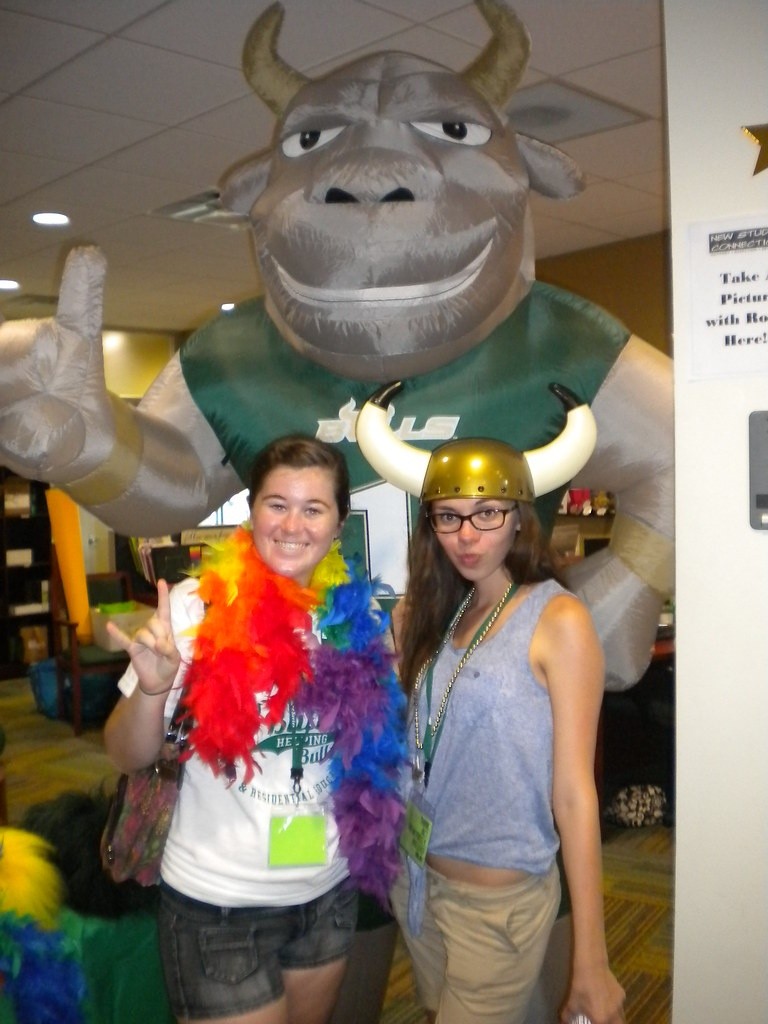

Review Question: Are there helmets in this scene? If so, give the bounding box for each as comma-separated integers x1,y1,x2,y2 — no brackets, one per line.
355,379,597,507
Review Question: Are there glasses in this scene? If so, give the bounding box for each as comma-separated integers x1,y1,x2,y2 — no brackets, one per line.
426,504,519,533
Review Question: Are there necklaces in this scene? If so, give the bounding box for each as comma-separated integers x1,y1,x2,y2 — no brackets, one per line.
413,579,510,750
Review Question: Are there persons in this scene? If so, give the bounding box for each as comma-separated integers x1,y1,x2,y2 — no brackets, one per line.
383,437,628,1024
103,435,408,1024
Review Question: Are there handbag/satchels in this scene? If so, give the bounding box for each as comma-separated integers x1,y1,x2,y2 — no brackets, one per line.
99,759,181,885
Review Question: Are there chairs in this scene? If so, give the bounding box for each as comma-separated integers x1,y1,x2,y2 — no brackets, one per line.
49,572,134,738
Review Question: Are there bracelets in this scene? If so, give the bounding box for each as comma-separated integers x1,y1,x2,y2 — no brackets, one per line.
138,683,172,696
